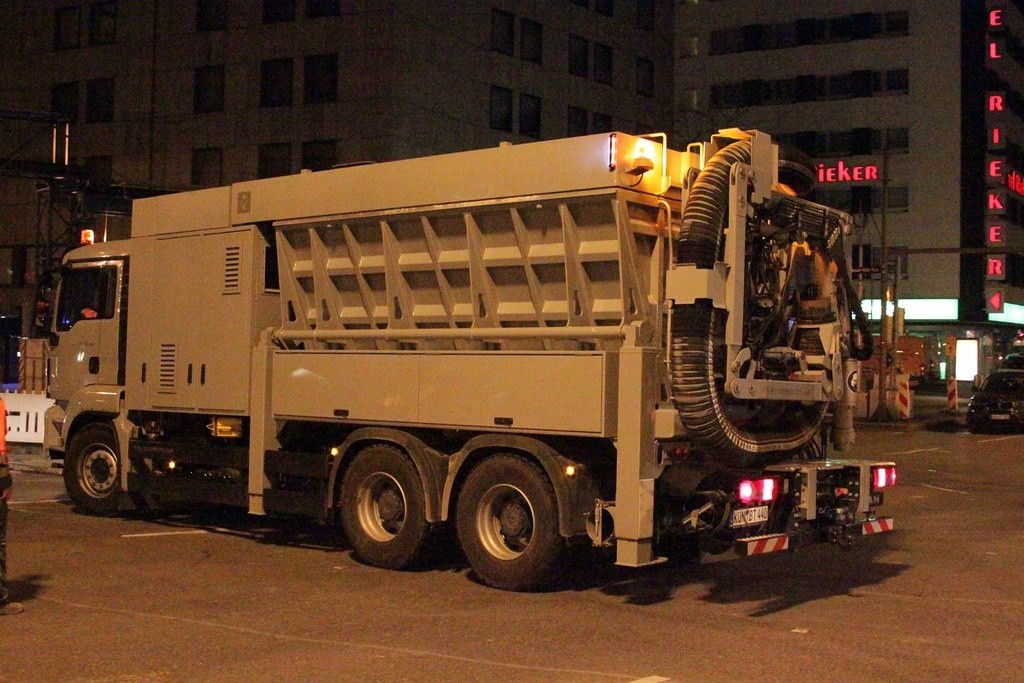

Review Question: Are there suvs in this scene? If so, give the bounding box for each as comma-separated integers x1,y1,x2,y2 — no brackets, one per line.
997,353,1024,372
965,370,1024,433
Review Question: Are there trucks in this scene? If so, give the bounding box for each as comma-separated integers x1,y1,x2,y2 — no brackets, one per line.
861,336,925,388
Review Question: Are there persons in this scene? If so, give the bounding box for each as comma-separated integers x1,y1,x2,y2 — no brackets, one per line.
0,398,24,615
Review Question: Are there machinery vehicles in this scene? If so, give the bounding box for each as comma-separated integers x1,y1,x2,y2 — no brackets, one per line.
27,128,898,594
